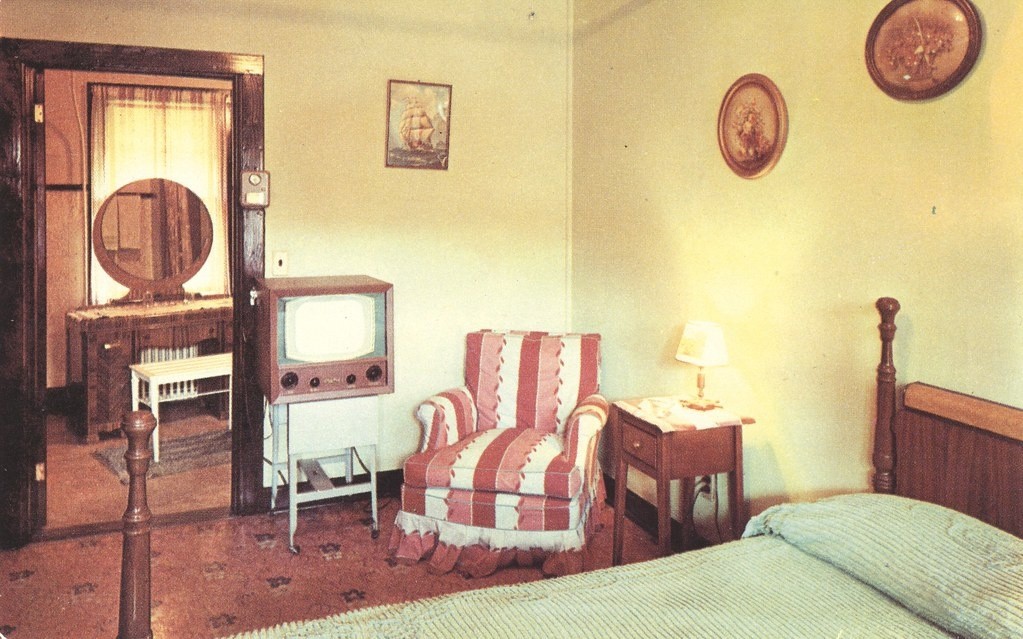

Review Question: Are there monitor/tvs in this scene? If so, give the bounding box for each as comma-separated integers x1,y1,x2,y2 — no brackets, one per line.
253,274,394,405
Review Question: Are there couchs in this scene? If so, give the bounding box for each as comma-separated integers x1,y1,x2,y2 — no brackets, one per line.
386,328,610,577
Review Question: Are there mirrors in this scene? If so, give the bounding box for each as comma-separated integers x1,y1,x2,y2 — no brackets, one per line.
94,177,213,292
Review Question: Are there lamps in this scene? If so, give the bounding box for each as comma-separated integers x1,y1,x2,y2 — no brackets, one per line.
676,322,730,411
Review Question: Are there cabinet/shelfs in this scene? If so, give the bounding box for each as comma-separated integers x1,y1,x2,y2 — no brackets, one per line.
65,296,233,443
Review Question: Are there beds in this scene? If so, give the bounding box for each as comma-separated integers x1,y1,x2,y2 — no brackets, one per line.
119,297,1023,639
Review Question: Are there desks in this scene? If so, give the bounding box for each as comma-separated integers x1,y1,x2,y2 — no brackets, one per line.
611,399,755,567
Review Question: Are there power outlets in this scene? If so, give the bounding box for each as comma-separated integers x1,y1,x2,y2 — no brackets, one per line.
702,474,716,499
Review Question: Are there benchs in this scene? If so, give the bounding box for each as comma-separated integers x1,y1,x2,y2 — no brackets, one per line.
128,353,233,462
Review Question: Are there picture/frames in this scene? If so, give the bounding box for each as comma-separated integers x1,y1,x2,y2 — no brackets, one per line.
385,79,452,169
716,73,789,182
865,0,981,104
